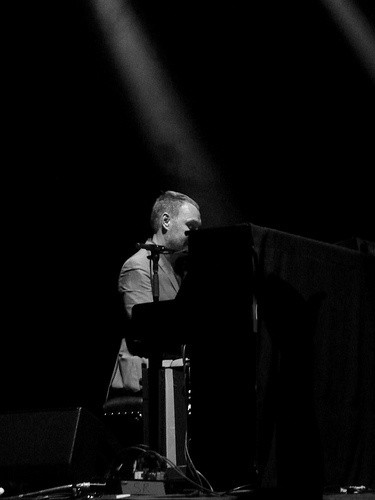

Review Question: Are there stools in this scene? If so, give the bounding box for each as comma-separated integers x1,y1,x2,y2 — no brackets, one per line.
103,396,143,446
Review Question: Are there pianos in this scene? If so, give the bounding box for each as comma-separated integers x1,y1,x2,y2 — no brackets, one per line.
126,220,375,491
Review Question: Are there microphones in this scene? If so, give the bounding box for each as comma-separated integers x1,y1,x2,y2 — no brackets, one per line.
173,244,188,254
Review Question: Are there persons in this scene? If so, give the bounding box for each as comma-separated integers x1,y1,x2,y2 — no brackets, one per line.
109,192,203,397
